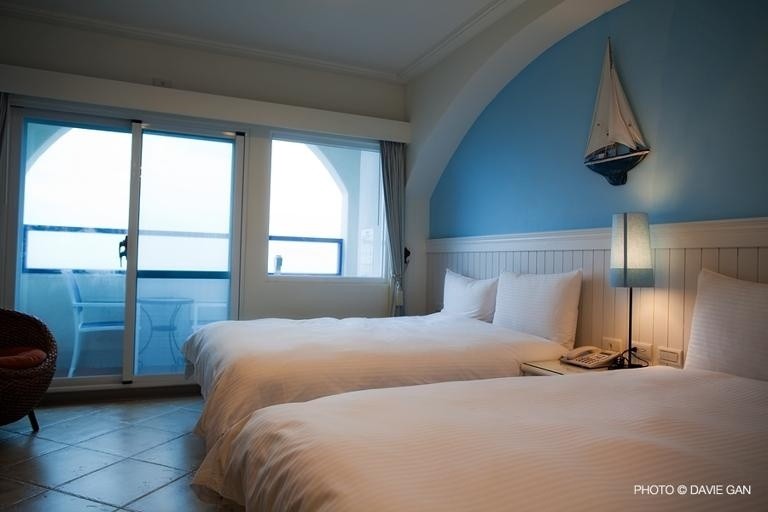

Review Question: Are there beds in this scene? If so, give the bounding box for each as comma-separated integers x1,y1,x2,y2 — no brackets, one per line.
192,312,768,511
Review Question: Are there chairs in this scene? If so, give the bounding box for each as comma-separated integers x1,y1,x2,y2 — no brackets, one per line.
0,307,58,431
60,267,140,380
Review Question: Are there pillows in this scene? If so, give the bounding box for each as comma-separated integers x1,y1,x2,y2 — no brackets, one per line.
683,266,768,381
440,266,583,348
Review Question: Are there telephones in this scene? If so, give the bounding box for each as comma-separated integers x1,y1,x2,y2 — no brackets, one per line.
560,345,620,369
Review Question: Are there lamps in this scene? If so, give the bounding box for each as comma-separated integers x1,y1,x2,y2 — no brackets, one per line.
608,212,655,371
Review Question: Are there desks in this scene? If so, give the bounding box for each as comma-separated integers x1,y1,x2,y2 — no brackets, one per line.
137,296,194,371
